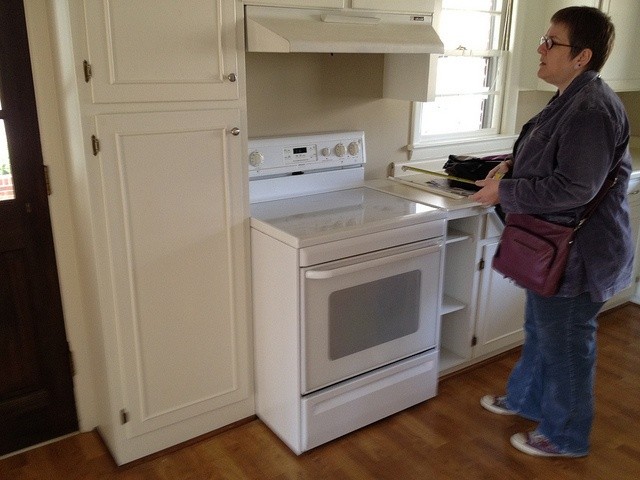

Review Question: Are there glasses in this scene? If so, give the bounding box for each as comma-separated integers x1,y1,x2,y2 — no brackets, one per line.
539,35,572,50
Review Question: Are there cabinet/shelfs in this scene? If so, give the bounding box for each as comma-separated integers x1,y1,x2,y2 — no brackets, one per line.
511,0,639,93
96,107,249,440
477,207,528,359
83,1,239,104
436,209,476,378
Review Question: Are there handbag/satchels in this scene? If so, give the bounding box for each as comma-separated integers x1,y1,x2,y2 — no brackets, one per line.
492,158,622,298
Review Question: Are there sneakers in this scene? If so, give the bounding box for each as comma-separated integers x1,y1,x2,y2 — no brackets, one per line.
480,394,515,416
510,428,589,457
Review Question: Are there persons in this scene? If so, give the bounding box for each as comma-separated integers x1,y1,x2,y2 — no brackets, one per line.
468,6,634,457
